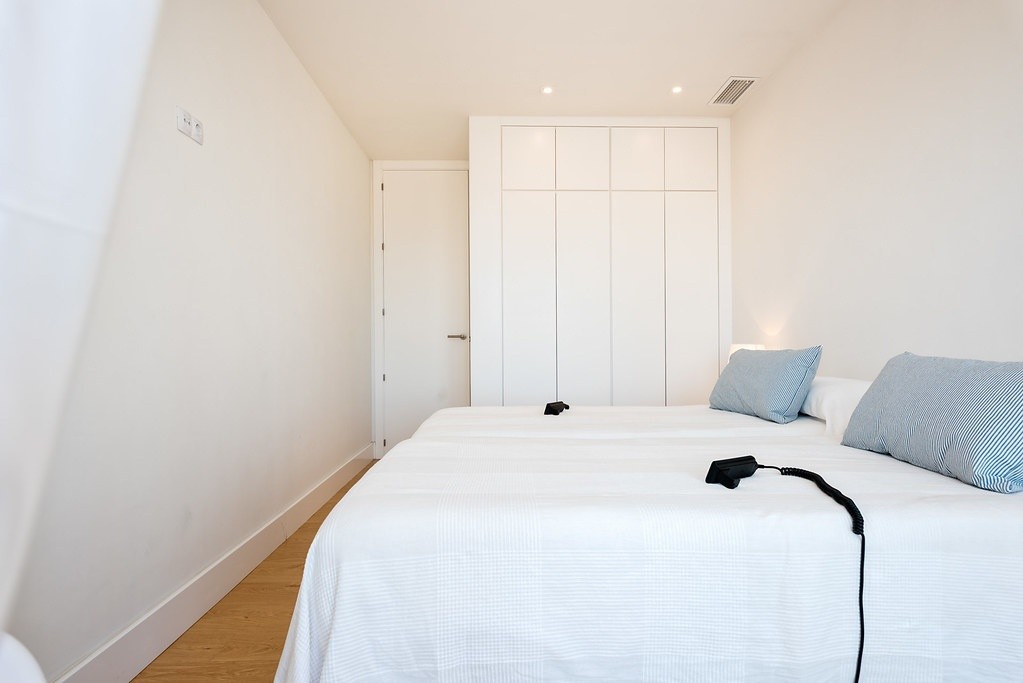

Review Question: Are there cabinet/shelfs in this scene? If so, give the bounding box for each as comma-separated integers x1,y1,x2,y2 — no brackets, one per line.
467,115,732,407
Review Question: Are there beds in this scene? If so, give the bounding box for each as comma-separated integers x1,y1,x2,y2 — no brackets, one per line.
272,440,1023,683
411,407,830,439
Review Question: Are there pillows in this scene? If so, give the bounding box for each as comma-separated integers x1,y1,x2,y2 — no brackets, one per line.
710,342,823,424
799,376,873,439
841,351,1023,493
